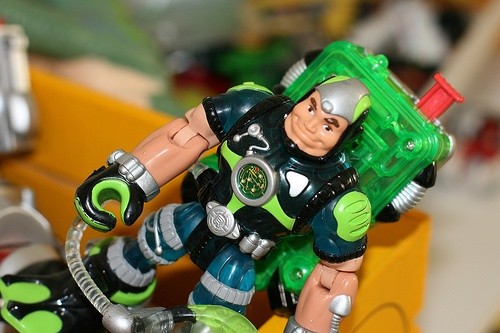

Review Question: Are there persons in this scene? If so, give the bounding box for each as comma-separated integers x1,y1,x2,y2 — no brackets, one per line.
0,73,379,333
